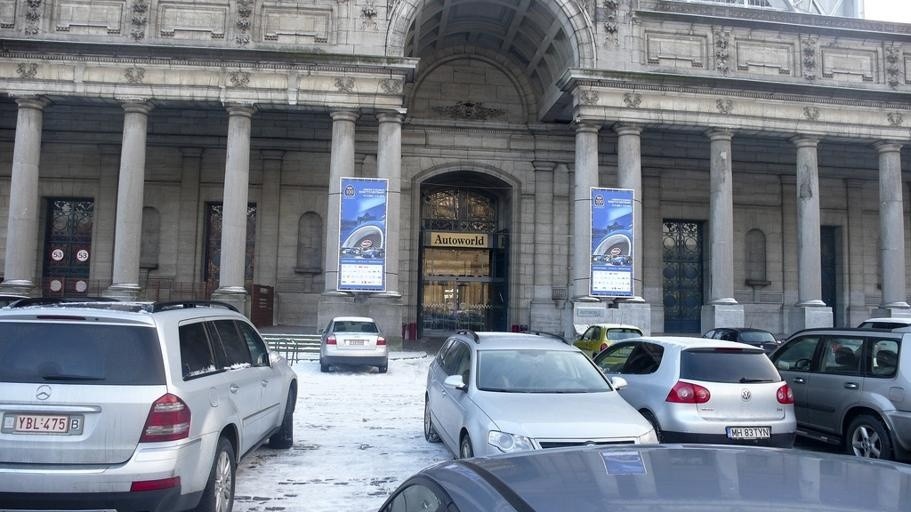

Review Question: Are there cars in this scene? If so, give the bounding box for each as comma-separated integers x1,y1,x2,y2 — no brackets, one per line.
834,317,910,352
378,444,911,512
0,295,118,309
591,337,799,448
317,316,388,372
340,245,383,258
592,254,631,265
574,323,646,367
703,327,778,358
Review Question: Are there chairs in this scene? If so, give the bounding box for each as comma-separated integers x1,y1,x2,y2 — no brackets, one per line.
825,347,897,378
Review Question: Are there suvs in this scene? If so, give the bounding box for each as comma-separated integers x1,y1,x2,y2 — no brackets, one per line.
423,327,660,463
0,294,297,510
769,327,909,463
